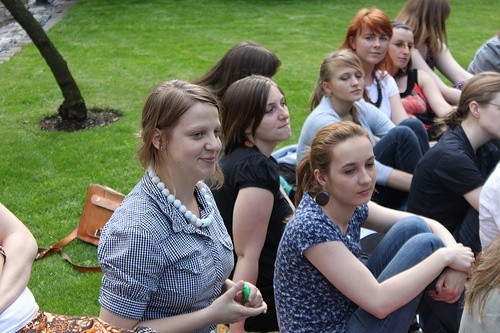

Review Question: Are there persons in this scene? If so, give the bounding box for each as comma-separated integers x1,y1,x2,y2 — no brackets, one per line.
98,79,268,333
273,121,475,333
192,41,281,104
341,0,500,333
295,48,430,211
204,75,295,333
0,202,135,333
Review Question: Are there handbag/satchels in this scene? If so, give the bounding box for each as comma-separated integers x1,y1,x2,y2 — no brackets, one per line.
77,183,127,247
